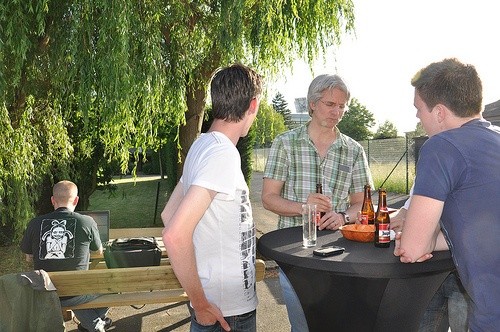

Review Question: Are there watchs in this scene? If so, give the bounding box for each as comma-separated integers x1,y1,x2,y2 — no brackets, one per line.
338,211,350,224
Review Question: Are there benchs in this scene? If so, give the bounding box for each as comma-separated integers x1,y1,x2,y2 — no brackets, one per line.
38,227,266,313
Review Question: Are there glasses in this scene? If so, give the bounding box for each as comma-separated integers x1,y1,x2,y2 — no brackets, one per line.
319,100,345,109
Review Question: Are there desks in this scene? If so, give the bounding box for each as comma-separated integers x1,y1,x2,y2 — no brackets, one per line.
89,236,166,268
257,220,459,332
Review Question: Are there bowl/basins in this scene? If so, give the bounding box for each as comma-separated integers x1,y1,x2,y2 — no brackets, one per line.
338,224,376,243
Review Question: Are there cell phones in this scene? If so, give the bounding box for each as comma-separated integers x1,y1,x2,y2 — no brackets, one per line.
313,246,345,256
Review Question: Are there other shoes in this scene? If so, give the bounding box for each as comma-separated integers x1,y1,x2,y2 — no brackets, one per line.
94,317,112,332
78,323,89,332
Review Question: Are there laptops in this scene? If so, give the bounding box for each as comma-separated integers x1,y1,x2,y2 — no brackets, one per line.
76,210,113,246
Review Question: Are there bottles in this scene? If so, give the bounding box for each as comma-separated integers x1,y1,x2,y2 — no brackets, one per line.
315,184,327,231
375,190,391,248
361,184,374,224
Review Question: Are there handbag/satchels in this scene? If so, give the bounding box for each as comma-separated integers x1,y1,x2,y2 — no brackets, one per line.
104,237,162,268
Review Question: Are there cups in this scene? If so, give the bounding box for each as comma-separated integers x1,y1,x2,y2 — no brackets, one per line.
302,204,317,247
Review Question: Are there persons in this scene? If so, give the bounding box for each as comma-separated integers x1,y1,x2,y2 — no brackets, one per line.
20,180,112,332
260,75,375,332
389,95,500,332
392,59,499,331
159,62,263,330
